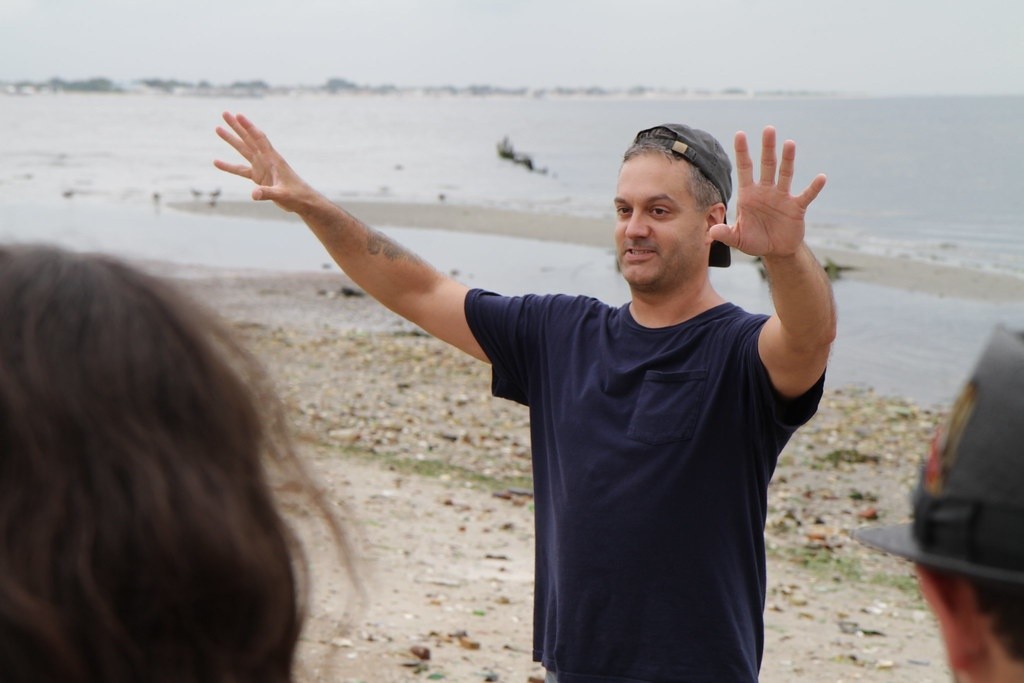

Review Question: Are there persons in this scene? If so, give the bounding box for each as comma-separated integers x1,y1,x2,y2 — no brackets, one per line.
1,242,303,683
215,108,835,683
855,319,1024,683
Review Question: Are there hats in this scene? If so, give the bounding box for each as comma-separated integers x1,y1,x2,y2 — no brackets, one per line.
632,122,732,268
853,322,1024,585
